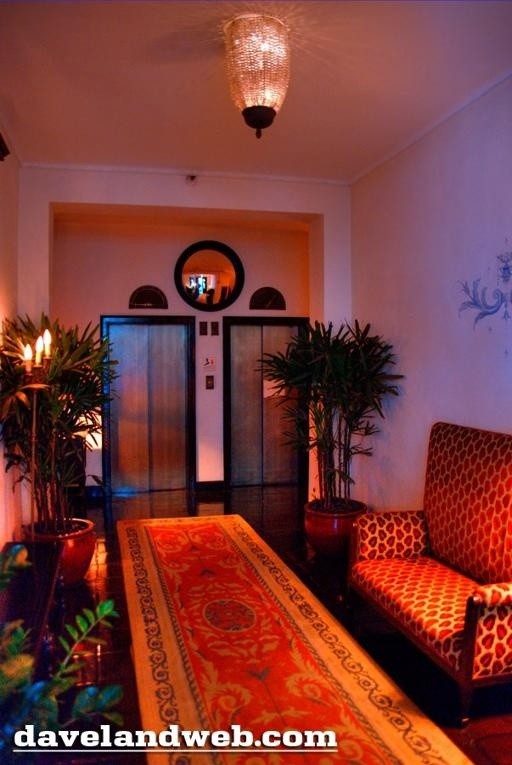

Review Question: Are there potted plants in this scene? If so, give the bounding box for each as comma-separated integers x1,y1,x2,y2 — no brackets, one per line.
0,314,121,585
256,321,406,554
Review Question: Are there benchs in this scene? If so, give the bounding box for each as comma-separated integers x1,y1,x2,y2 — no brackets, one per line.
349,421,512,726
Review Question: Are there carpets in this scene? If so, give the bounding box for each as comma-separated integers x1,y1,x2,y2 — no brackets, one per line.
116,513,477,765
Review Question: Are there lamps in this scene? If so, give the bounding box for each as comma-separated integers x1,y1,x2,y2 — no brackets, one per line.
221,12,291,138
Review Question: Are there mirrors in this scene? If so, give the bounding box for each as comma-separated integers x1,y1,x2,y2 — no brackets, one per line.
174,240,245,312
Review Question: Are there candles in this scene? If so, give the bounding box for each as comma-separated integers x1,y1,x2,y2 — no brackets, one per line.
35,336,44,365
24,343,33,373
43,329,52,357
